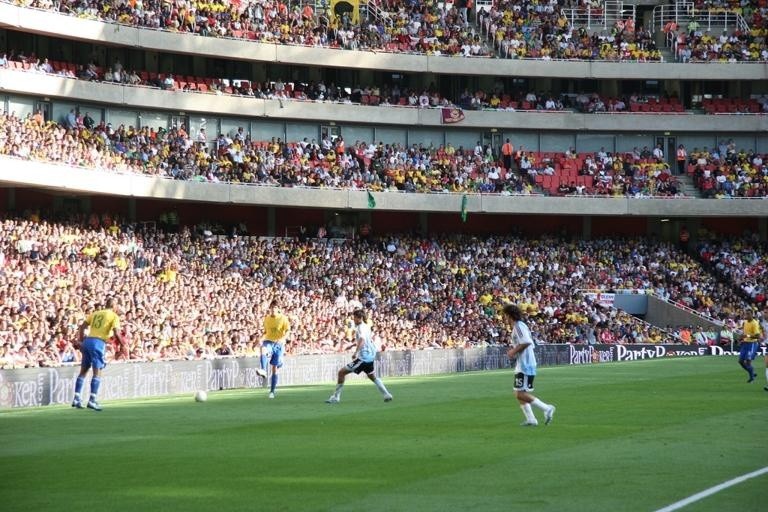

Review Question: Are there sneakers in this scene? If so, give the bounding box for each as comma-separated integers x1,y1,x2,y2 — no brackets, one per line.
269,392,274,399
72,399,86,409
87,400,102,411
520,420,539,426
256,369,267,379
383,394,392,401
325,397,340,403
544,404,556,426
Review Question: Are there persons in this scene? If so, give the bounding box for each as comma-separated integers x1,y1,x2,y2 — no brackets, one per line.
320,309,394,404
254,299,291,400
67,298,128,411
501,306,557,429
0,1,768,371
756,304,768,393
733,308,764,384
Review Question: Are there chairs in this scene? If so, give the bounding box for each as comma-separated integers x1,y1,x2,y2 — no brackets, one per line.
467,150,718,192
500,93,531,110
630,96,760,113
2,59,233,94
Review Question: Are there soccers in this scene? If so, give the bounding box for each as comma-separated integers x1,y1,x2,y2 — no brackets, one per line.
195,390,206,401
